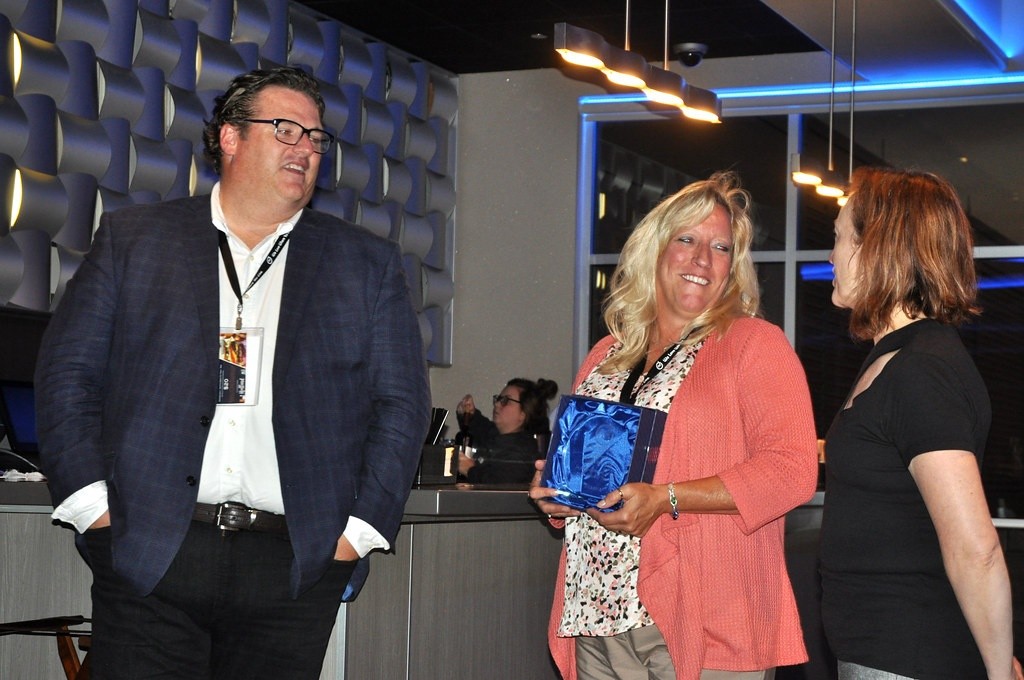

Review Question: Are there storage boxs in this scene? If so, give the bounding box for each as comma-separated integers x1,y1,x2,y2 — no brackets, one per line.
539,393,668,515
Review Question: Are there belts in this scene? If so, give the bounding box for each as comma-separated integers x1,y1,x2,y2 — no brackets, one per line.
191,502,288,538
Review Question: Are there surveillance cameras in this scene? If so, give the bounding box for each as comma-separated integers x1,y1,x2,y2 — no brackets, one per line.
672,43,706,66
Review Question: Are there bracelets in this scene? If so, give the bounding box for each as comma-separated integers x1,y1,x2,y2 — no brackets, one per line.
668,484,680,520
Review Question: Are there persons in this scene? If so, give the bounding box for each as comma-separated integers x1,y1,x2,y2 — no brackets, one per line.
455,378,560,486
820,166,1024,679
31,64,434,680
529,166,820,679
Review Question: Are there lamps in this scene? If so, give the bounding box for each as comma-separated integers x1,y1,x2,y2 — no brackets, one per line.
790,0,858,208
553,0,725,125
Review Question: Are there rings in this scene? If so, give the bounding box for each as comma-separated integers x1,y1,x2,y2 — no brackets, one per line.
618,489,624,500
548,513,552,519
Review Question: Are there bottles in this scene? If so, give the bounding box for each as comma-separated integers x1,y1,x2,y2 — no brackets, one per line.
452,412,474,483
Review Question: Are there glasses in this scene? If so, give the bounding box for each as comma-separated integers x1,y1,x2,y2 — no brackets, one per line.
230,118,336,156
493,395,522,405
